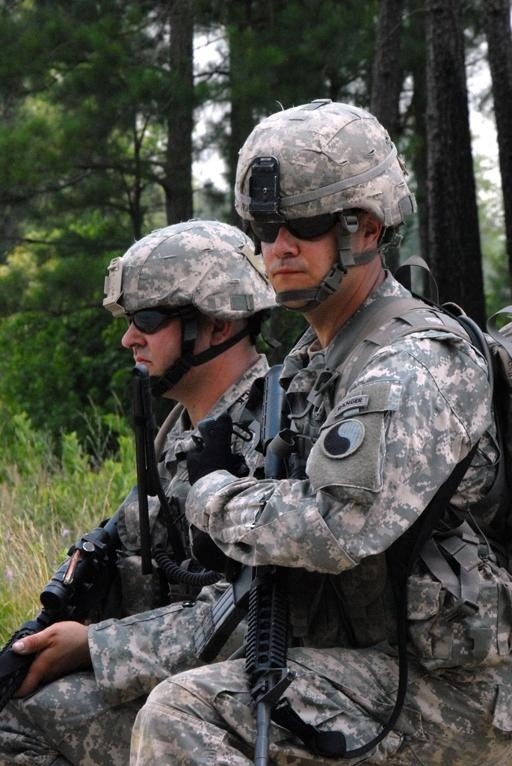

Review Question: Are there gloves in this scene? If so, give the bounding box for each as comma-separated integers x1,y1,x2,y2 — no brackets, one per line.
186,413,247,487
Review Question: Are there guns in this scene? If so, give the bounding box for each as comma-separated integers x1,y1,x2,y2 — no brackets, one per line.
190,364,296,766
0,485,140,716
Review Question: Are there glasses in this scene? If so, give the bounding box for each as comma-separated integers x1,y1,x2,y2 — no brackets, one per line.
121,304,183,335
246,210,348,240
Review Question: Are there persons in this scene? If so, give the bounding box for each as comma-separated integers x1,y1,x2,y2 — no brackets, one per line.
0,216,287,766
131,95,512,766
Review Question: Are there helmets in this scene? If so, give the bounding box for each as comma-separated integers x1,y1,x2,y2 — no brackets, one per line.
102,217,283,320
234,100,418,232
